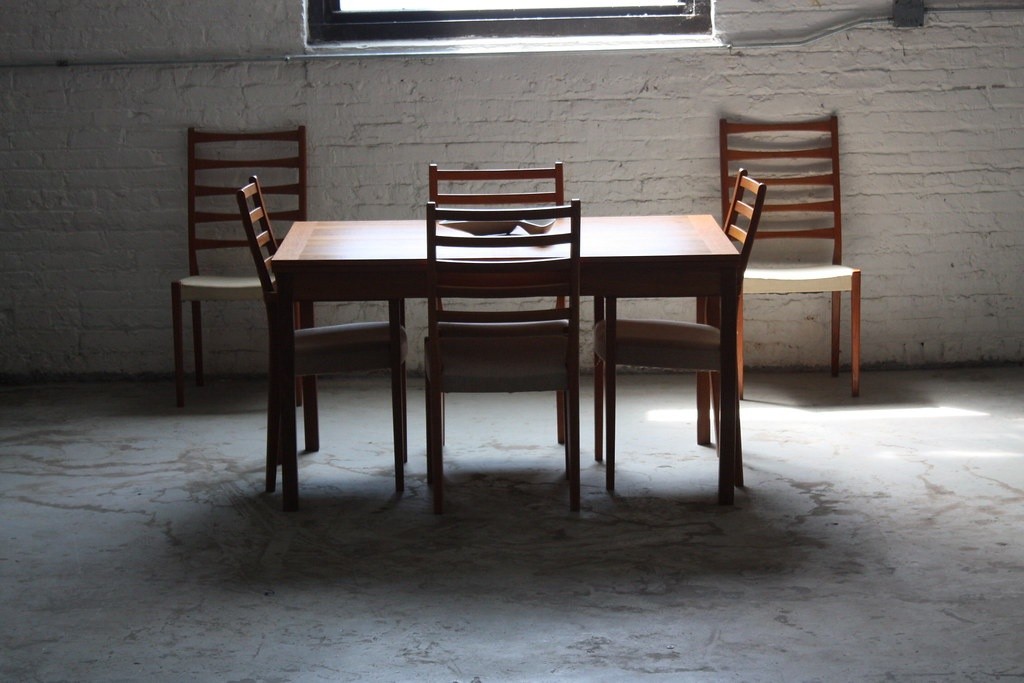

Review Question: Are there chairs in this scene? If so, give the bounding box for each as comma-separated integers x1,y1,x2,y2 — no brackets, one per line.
720,117,860,400
237,176,408,496
170,126,307,408
425,162,581,512
593,168,766,493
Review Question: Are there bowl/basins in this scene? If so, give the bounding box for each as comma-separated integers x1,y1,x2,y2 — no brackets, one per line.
519,219,559,235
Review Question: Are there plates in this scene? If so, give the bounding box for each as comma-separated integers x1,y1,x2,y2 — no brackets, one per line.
437,219,521,237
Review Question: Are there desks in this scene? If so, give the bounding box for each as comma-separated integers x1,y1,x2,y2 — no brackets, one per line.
272,214,741,513
